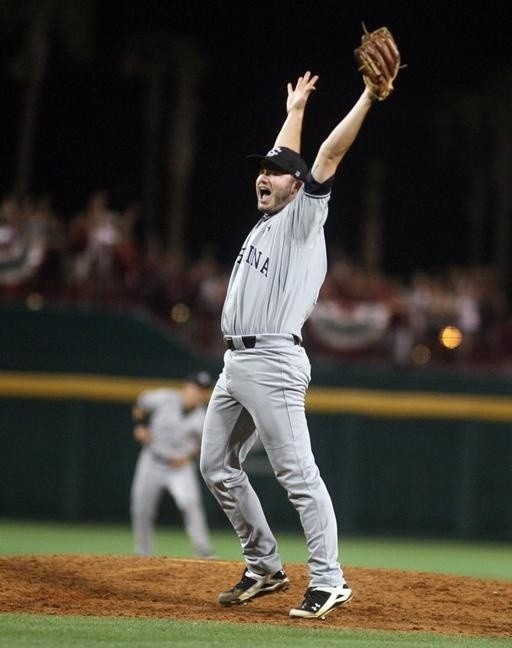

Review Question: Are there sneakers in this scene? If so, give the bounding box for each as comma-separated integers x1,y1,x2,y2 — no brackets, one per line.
289,578,353,618
218,565,288,605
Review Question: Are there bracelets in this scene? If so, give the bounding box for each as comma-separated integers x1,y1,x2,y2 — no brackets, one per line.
132,420,144,425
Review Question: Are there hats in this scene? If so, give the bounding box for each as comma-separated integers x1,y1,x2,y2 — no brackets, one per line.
247,147,308,183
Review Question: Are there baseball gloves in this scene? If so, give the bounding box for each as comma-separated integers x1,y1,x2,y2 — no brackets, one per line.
354,20,407,101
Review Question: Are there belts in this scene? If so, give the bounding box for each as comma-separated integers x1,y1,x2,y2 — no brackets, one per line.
227,334,299,351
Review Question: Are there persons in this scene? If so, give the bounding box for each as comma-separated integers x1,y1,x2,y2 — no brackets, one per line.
305,261,512,369
199,26,402,619
129,370,216,561
1,191,225,368
